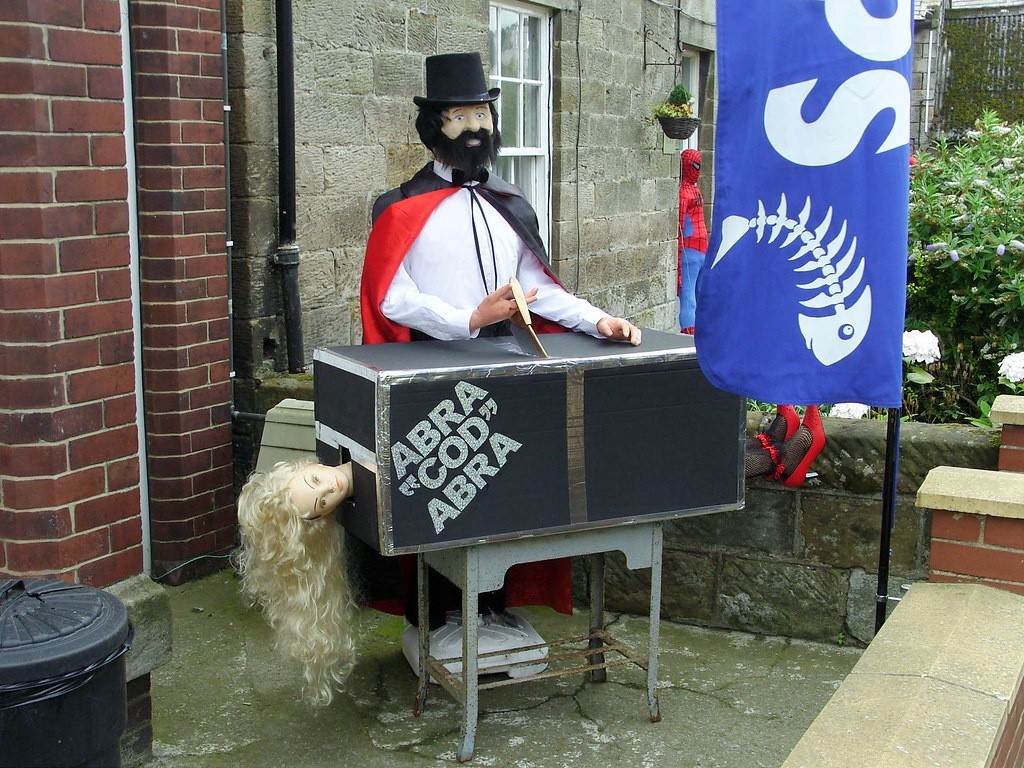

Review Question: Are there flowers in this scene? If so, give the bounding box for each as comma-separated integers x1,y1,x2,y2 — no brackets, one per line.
643,85,696,128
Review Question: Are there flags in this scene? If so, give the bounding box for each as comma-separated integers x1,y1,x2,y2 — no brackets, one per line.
691,0,915,407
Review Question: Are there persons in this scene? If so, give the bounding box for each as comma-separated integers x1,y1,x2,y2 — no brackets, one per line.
677,149,716,337
237,399,830,711
363,52,643,627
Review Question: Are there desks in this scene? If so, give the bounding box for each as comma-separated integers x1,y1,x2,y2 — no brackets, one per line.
411,522,662,763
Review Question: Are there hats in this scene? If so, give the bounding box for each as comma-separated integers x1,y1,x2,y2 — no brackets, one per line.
413,52,500,108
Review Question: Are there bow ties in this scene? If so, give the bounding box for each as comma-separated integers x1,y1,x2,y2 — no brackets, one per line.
451,165,489,187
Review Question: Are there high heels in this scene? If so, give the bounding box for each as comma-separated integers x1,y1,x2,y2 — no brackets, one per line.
754,404,801,447
762,404,827,487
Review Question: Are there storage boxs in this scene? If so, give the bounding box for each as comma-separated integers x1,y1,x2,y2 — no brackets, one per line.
312,328,746,556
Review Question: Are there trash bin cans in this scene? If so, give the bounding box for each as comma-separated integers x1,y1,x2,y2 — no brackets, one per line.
0,575,137,768
